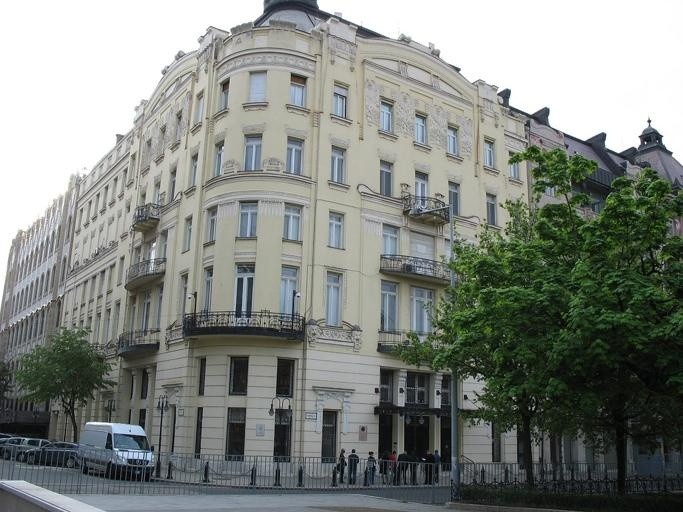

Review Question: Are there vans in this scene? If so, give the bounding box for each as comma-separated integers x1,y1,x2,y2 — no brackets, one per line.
79,421,155,481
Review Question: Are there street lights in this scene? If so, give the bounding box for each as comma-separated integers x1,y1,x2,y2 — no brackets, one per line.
409,205,460,501
268,396,293,486
156,394,168,479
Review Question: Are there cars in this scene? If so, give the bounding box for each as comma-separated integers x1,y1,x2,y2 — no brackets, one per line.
0,433,79,468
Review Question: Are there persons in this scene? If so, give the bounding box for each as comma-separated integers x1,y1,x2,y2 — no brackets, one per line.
346,448,359,483
366,449,441,485
339,448,347,483
